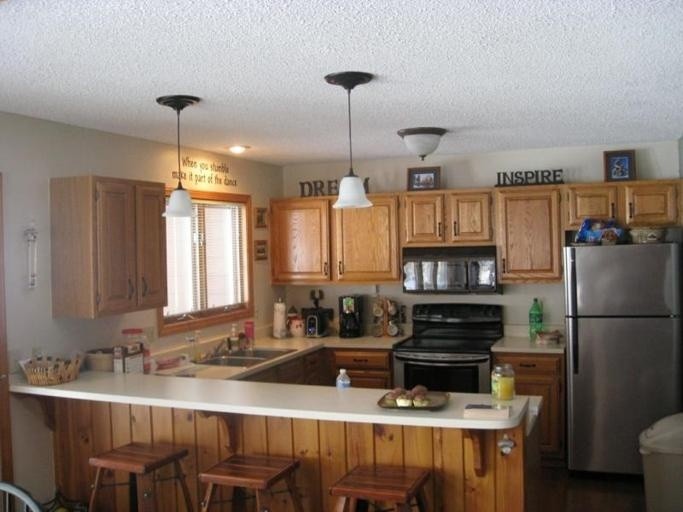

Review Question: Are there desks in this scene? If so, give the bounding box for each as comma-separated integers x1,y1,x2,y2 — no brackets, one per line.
5,371,530,512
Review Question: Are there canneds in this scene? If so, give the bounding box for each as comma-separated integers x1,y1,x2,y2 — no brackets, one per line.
490,363,516,400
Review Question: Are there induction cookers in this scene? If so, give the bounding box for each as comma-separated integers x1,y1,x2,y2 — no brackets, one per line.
392,333,499,363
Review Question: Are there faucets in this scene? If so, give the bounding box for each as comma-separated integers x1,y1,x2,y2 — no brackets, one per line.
211,335,232,358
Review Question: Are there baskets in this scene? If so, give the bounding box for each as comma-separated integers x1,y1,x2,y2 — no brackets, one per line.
19,351,85,385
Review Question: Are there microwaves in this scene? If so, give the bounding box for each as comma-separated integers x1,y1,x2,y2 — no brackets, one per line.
400,253,505,297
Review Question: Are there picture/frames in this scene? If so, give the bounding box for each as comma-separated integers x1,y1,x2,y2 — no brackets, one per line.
405,166,441,191
602,150,637,181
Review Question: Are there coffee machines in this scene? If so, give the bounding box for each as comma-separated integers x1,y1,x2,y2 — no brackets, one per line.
337,293,366,339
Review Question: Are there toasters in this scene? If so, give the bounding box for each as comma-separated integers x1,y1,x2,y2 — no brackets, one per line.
304,310,330,339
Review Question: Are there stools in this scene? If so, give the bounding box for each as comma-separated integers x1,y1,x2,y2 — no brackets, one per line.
87,440,197,511
193,451,304,511
326,463,431,511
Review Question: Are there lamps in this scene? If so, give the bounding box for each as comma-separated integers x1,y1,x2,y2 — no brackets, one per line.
395,125,447,162
156,95,203,219
322,70,375,212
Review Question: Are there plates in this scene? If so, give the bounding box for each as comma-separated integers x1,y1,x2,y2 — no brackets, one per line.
377,391,450,410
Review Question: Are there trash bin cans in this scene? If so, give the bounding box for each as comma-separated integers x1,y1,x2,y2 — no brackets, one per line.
638,413,683,512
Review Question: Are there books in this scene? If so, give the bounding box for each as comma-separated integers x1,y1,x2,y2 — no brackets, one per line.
462,403,510,420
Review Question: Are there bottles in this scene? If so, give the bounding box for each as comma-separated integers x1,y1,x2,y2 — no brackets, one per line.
499,362,515,400
490,366,502,399
529,299,544,339
229,323,238,352
121,328,151,374
273,296,289,339
335,368,351,402
244,318,256,351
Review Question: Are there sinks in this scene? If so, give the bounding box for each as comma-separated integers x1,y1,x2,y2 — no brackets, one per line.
190,356,266,369
226,345,299,360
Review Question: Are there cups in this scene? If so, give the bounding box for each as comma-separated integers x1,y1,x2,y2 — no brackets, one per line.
386,298,396,316
368,319,386,338
371,298,383,317
386,320,400,336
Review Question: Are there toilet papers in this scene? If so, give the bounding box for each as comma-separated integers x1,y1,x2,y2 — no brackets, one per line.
272,301,287,338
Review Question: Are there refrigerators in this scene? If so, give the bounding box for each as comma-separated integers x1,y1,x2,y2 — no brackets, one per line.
560,241,682,477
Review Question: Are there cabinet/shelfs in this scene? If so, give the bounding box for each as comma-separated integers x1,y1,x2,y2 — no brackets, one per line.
401,190,492,250
265,193,402,288
49,174,171,322
325,349,391,389
561,178,682,233
493,354,565,471
493,182,560,284
241,347,326,385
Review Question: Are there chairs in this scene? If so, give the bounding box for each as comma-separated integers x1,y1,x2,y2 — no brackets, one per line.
0,478,38,511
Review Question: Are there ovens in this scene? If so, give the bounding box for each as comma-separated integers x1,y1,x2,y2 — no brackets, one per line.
392,359,490,394
